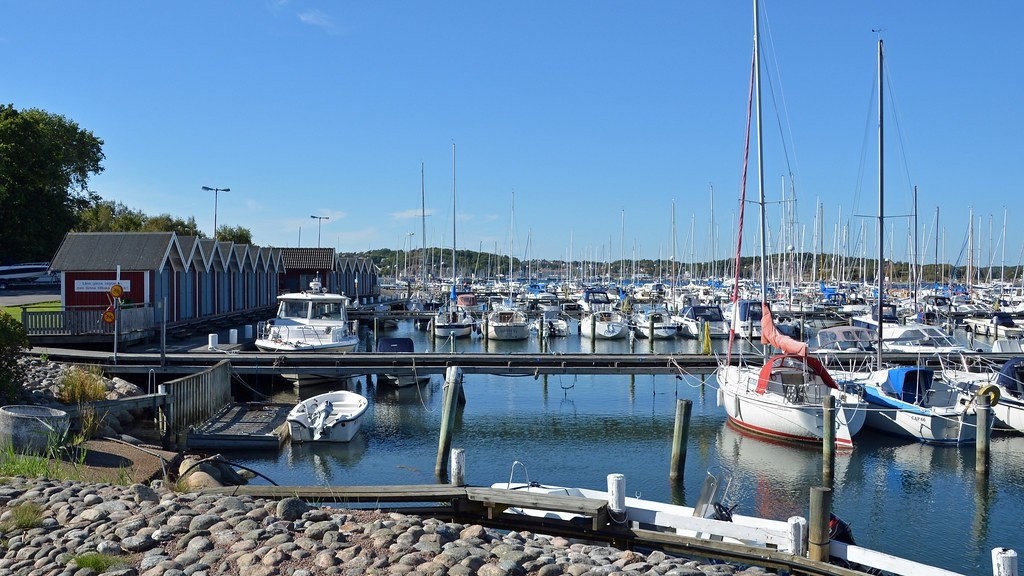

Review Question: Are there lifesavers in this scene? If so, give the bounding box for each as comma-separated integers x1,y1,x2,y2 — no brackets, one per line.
978,385,1000,407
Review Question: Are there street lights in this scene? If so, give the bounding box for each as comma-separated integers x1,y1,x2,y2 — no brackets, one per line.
310,215,329,249
202,185,231,239
407,232,414,272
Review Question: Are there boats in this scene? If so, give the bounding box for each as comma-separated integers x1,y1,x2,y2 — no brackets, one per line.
254,271,360,389
491,463,858,563
377,336,432,388
939,352,1024,435
287,388,370,441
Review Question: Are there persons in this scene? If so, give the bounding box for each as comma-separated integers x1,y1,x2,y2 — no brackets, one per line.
917,309,924,324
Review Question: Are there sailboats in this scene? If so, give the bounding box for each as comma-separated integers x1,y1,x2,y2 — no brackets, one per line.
826,39,996,448
716,0,872,453
378,141,1024,369
